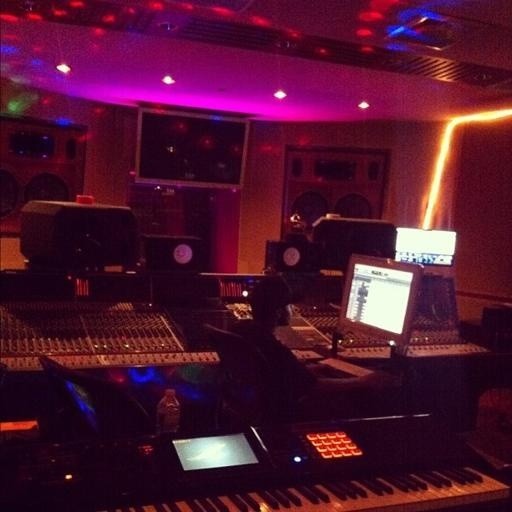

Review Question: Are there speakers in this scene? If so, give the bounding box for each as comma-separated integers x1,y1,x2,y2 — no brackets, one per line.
265,239,319,271
312,217,396,270
143,234,204,273
18,200,139,266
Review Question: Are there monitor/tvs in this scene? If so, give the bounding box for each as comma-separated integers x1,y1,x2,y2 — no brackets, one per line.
395,227,457,267
133,107,252,191
340,254,425,345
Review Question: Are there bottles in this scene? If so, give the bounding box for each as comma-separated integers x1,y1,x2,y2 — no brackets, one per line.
156,388,180,441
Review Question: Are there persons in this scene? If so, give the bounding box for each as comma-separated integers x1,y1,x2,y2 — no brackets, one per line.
221,275,401,425
